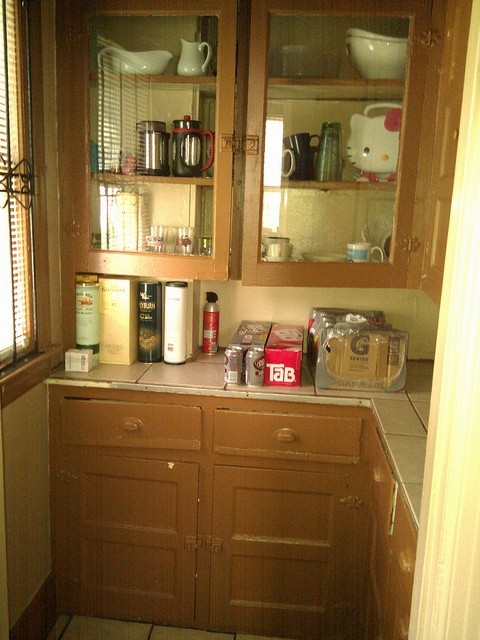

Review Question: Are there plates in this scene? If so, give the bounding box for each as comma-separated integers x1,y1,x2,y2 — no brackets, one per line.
303,252,348,261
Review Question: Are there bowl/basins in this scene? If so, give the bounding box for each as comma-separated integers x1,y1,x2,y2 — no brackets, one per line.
277,45,343,81
344,27,408,80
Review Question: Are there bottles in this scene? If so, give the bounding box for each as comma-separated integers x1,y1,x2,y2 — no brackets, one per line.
202,292,222,354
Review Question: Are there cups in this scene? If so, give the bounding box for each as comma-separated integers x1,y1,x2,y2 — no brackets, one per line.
346,243,384,263
283,132,320,179
134,120,170,179
174,227,195,254
267,236,293,261
148,226,167,254
198,236,211,257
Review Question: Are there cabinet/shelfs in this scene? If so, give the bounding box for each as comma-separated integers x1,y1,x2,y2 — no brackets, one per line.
421,0,471,301
59,397,203,629
54,0,432,288
365,425,417,639
209,408,365,639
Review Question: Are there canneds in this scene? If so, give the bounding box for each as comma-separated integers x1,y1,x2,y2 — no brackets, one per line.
223,346,244,387
245,348,264,387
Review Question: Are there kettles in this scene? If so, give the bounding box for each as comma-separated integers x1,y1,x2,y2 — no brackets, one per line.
171,115,214,177
